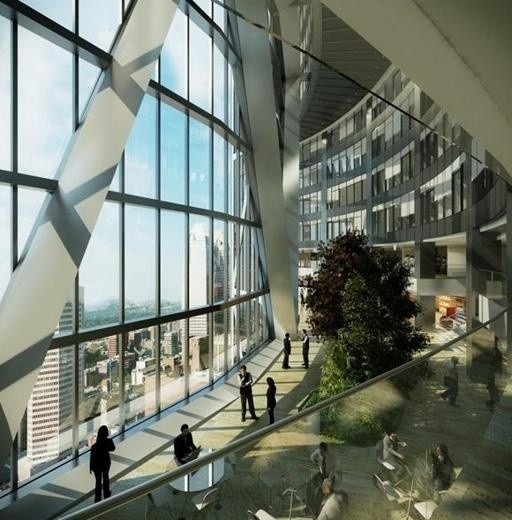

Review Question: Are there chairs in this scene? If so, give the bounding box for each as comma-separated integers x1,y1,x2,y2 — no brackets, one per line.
370,439,463,513
180,487,223,520
256,465,288,506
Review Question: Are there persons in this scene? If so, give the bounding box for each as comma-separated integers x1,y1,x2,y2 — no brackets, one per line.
428,441,454,491
89,424,116,503
311,441,339,496
236,364,260,422
448,358,462,408
315,477,342,520
382,423,412,486
266,376,277,426
281,332,291,369
174,423,198,467
441,355,459,402
486,366,502,412
296,328,310,369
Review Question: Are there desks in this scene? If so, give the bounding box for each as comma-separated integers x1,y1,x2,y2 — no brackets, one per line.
165,448,225,493
